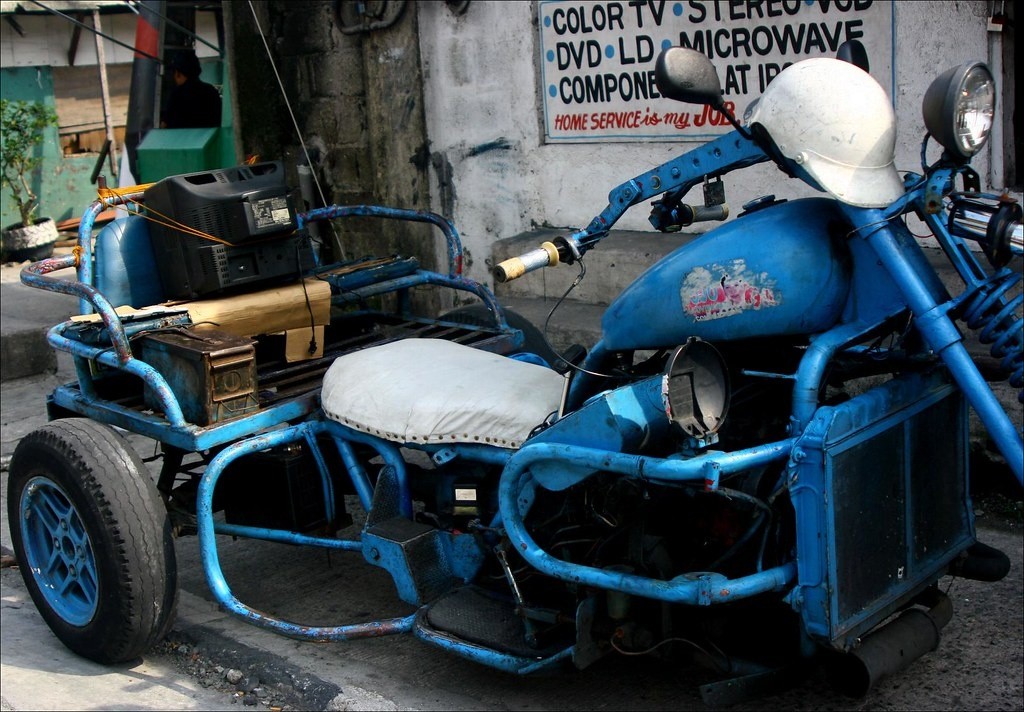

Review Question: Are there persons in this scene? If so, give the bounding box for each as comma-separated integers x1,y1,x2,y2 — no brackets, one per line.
159,51,222,130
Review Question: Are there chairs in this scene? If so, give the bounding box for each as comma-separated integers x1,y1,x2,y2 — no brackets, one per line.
19,181,523,453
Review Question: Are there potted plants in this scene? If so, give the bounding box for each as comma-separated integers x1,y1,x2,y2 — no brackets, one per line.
0,96,58,262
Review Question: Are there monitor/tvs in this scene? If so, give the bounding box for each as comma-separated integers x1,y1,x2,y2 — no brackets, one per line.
144,161,317,300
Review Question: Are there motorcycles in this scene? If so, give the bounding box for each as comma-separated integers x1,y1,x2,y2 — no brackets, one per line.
4,35,1024,712
